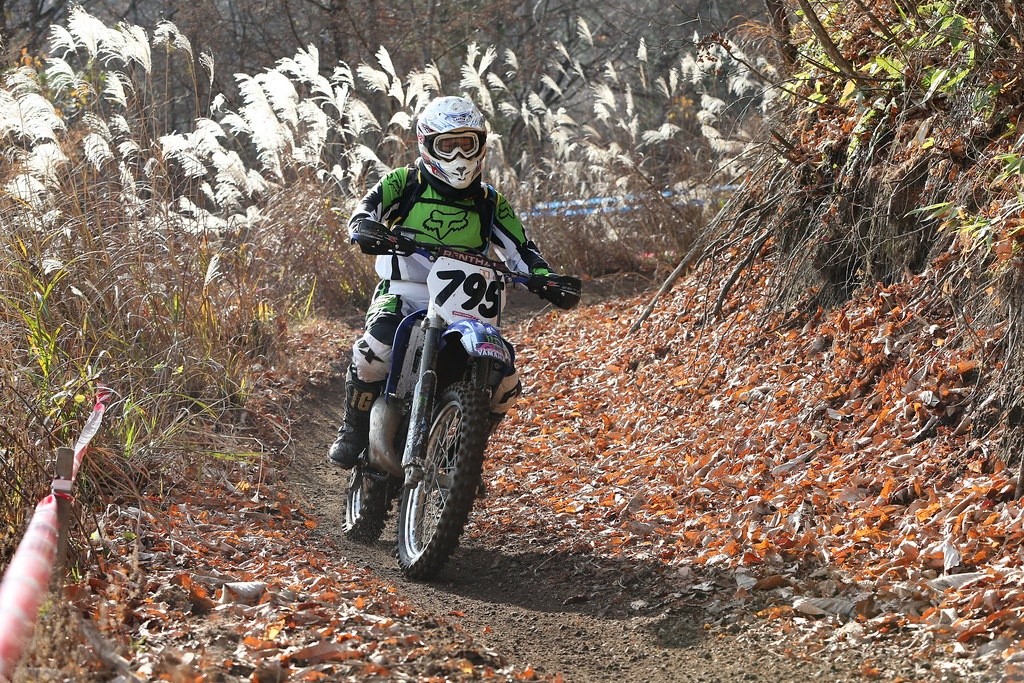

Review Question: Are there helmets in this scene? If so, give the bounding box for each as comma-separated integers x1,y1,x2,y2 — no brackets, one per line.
415,96,488,189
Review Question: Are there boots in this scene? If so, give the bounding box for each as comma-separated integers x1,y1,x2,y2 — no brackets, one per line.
327,361,387,469
475,475,488,499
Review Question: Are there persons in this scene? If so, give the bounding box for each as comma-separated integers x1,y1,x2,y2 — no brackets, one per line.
327,97,562,498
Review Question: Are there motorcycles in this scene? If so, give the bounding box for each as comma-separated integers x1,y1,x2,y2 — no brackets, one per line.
340,227,566,580
